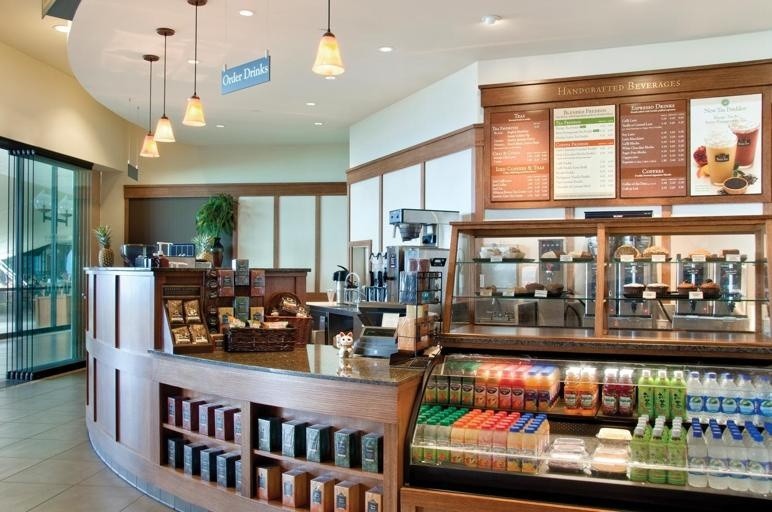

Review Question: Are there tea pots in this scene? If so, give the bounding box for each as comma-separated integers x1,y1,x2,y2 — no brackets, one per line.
344,282,361,304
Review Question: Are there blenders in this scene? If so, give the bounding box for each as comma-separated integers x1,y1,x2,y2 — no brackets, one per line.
331,264,352,303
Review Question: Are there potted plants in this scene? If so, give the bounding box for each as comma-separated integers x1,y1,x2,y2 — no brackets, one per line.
196,190,240,266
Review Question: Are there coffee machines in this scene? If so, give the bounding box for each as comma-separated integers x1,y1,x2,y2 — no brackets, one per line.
386,209,459,300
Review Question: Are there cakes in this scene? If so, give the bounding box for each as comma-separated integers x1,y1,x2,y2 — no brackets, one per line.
479,245,594,260
624,279,723,297
478,281,568,298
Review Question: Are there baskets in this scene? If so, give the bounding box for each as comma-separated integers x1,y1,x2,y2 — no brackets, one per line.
223,324,296,351
265,311,311,345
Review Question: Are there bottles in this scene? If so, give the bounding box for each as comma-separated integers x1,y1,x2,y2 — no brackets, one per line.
428,361,553,413
409,407,551,474
562,363,772,499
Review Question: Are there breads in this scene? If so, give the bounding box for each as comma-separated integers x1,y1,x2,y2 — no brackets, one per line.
612,245,745,262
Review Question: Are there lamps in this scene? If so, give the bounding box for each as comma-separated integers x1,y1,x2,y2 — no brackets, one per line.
180,0,209,128
313,1,346,77
139,27,176,157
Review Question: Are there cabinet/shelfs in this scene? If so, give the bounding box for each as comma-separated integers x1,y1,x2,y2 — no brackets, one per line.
150,380,398,510
441,211,772,345
399,343,770,510
